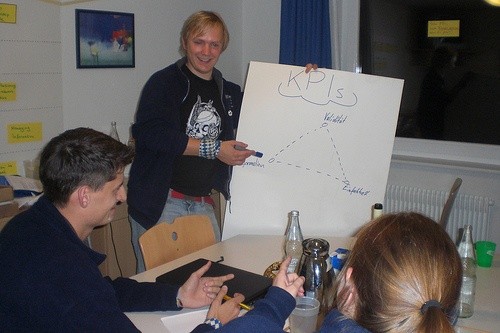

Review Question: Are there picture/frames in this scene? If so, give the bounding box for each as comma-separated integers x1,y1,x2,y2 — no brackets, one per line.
75,9,135,69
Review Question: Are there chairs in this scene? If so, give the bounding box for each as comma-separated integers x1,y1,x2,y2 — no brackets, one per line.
138,215,217,271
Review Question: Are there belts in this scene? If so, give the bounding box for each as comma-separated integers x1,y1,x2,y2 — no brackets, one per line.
170,190,215,209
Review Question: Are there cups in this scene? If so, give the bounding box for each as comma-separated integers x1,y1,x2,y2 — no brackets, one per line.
474,240,497,267
288,296,320,333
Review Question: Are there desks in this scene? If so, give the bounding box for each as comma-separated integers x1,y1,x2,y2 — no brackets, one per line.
123,234,500,333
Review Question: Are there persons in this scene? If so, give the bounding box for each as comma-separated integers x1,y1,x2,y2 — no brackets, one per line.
0,127,246,333
127,11,317,274
207,212,463,333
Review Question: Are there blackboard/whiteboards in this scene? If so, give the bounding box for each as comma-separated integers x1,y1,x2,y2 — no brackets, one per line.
220,60,404,243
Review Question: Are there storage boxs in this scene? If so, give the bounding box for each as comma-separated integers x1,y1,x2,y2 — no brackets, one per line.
91,189,220,281
0,185,20,231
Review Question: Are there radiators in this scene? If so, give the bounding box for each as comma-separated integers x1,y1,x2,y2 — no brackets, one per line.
385,184,495,243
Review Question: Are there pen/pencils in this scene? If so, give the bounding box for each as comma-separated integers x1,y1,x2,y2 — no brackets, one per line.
223,294,250,310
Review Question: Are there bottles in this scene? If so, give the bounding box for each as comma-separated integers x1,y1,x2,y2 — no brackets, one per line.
373,202,383,218
283,211,304,273
451,223,476,318
298,239,330,316
109,121,120,142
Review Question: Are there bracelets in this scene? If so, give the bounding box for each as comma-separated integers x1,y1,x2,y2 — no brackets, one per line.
176,293,182,310
199,137,220,161
206,318,222,329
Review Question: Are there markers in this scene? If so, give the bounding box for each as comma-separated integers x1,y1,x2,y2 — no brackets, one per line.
233,144,264,159
